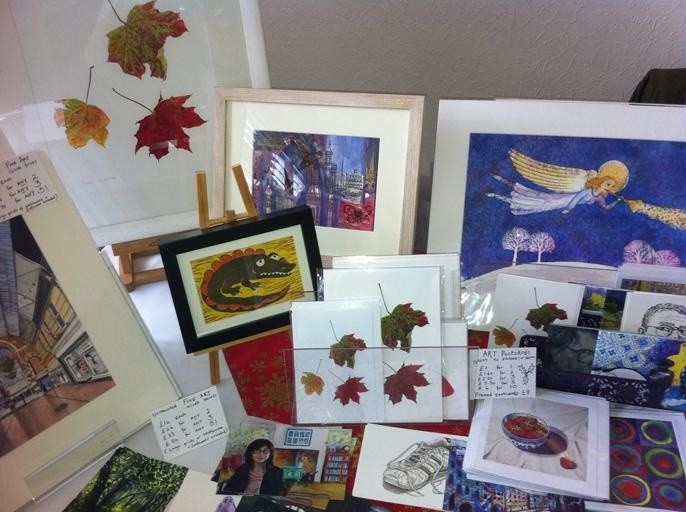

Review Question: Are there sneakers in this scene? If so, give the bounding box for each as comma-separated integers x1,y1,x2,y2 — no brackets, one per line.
383,435,454,496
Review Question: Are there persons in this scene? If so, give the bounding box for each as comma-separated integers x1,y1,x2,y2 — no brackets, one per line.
636,301,686,340
548,325,598,375
484,174,625,216
219,439,284,496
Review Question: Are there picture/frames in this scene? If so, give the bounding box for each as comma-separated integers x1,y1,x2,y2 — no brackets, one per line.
427,97,686,284
157,204,325,356
0,0,273,249
0,147,182,511
211,83,428,262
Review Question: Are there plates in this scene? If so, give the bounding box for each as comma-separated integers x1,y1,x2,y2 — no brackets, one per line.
514,426,569,455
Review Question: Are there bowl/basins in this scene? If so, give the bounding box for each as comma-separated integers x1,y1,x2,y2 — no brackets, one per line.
501,411,549,449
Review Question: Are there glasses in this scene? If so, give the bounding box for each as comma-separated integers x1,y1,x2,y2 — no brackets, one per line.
647,325,686,339
249,447,273,456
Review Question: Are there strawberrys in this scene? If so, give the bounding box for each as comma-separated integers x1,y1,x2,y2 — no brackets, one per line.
503,414,546,439
560,455,577,469
